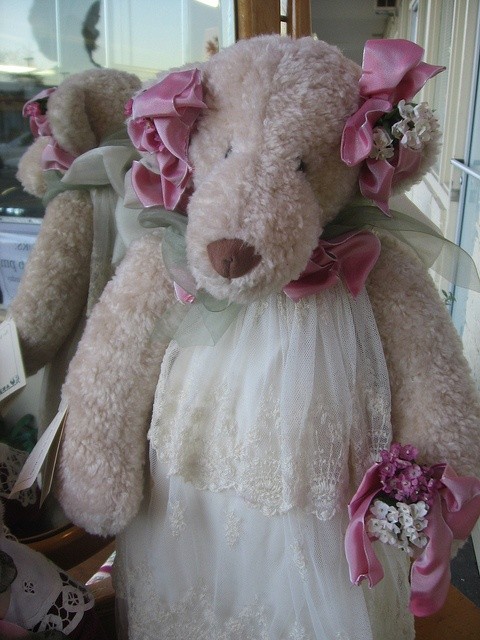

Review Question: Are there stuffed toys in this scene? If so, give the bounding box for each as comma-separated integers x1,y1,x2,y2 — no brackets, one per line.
54,32,480,638
1,67,144,443
